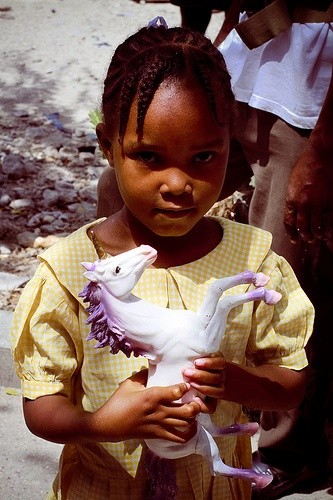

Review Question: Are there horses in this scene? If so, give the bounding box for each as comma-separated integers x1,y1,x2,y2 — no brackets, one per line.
77,244,282,490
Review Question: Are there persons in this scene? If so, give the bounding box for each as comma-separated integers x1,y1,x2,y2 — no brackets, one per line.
9,26,316,500
94,0,333,500
